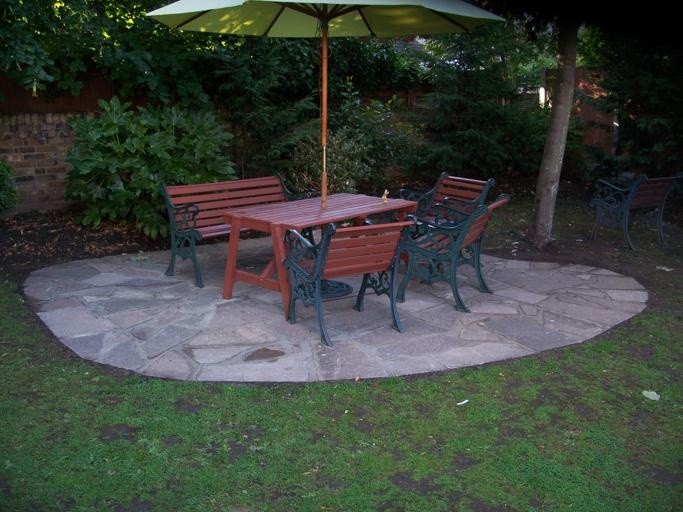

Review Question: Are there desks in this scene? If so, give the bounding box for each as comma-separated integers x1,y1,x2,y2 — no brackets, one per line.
223,192,418,319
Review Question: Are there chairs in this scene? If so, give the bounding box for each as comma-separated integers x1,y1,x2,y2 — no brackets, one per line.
589,173,683,252
388,172,495,286
281,214,418,346
395,194,511,313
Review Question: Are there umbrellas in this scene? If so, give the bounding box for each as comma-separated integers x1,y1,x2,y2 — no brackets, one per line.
143,1,505,209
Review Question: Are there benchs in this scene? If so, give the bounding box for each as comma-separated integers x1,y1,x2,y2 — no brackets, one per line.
160,170,302,288
